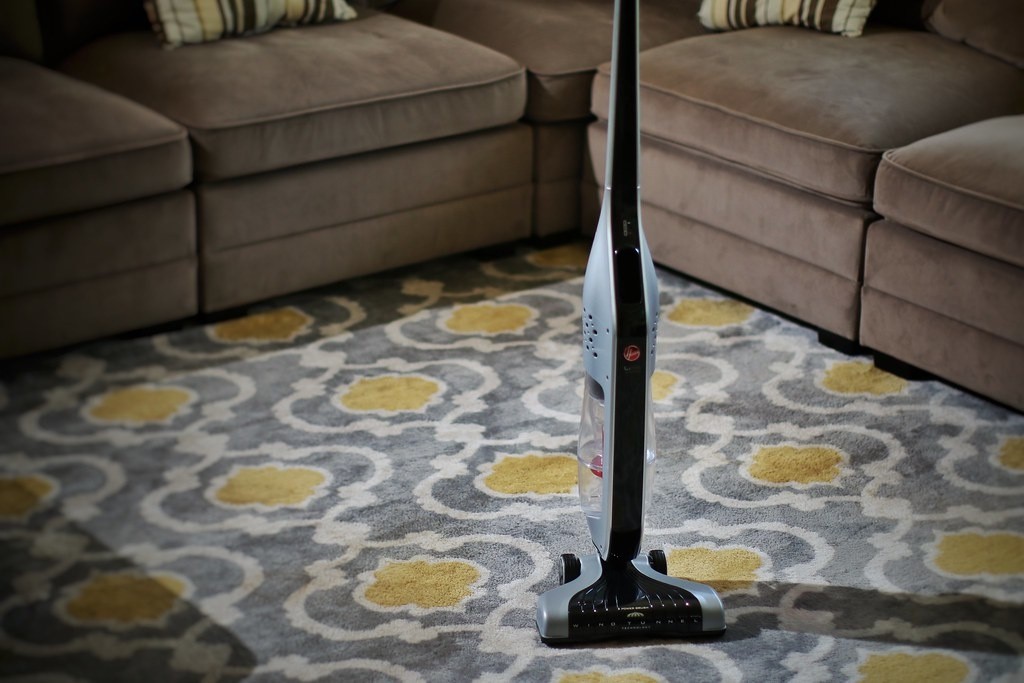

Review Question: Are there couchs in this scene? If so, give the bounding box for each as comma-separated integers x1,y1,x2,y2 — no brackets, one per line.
1,1,1024,416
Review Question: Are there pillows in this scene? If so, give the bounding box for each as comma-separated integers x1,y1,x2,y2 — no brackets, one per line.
145,0,358,52
696,0,877,38
